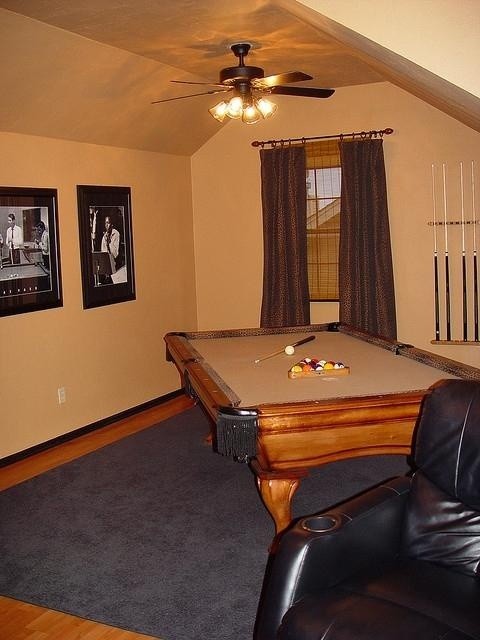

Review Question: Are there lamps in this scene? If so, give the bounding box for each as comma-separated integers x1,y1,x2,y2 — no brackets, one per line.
210,76,276,129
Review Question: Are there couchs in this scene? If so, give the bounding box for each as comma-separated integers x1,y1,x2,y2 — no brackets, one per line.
249,375,480,638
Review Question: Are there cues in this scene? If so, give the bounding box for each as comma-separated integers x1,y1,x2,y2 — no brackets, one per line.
255,336,315,363
432,161,478,342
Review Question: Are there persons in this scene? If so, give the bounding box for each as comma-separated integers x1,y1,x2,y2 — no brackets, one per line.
101,215,120,265
33,221,49,270
5,213,23,264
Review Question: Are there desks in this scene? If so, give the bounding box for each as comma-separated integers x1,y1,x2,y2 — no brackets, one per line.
162,322,480,551
0,263,50,294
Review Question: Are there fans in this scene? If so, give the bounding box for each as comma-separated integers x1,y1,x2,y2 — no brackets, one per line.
148,39,337,109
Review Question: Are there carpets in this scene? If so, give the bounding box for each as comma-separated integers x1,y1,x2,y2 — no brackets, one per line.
0,408,411,640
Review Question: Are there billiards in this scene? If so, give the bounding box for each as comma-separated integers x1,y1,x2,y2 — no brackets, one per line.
291,358,345,372
285,346,294,355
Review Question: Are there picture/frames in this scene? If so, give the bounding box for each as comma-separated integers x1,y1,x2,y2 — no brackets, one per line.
0,185,65,316
74,183,137,311
